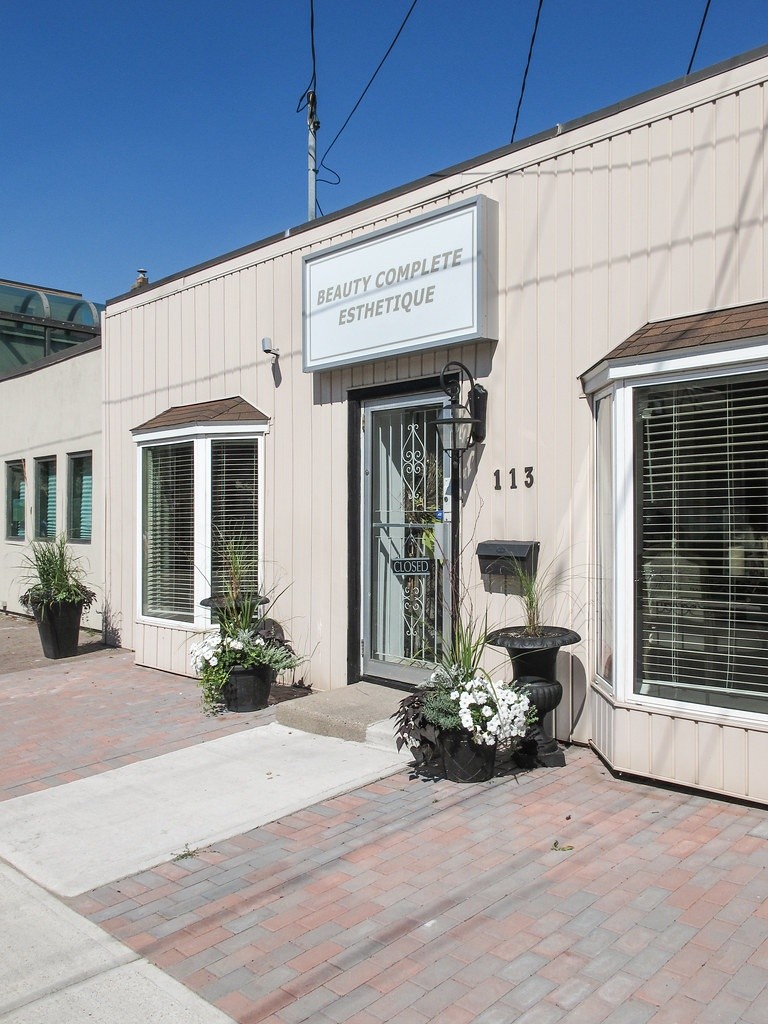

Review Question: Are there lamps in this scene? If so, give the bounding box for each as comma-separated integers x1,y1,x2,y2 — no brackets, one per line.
427,361,487,468
262,337,281,357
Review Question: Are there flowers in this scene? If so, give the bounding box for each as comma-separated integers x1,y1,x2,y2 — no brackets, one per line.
396,568,533,753
188,591,309,716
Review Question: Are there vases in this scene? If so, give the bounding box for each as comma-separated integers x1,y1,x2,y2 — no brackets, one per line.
440,724,497,781
220,660,273,711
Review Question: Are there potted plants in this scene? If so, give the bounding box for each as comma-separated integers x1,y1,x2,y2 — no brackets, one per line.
194,523,270,640
16,530,94,659
488,545,581,767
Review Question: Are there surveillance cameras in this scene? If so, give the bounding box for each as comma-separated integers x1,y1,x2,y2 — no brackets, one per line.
262,338,271,353
